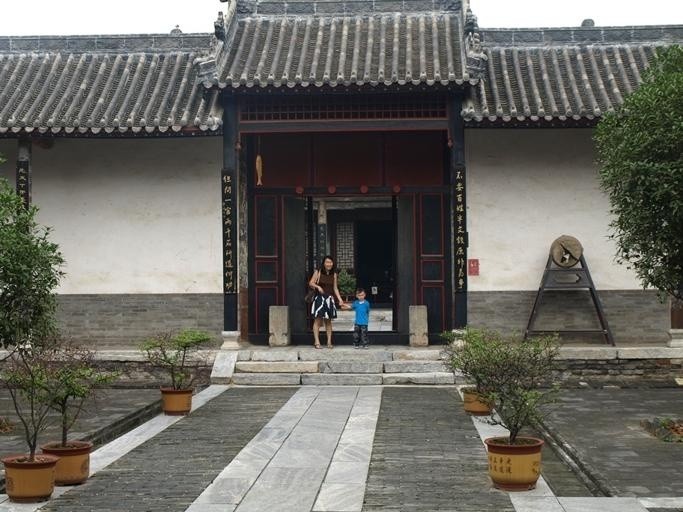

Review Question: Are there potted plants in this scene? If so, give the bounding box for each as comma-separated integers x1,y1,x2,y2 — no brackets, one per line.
0,338,137,502
338,268,356,311
137,328,216,416
439,325,563,491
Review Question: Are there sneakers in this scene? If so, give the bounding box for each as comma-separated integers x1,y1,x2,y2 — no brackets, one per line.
354,343,369,349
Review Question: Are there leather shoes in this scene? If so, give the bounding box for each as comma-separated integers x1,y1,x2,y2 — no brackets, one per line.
314,342,333,349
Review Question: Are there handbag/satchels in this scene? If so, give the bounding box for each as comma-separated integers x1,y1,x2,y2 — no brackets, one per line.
305,290,317,304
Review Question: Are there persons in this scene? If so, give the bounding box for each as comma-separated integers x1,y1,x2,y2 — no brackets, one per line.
307,255,343,349
339,288,371,350
